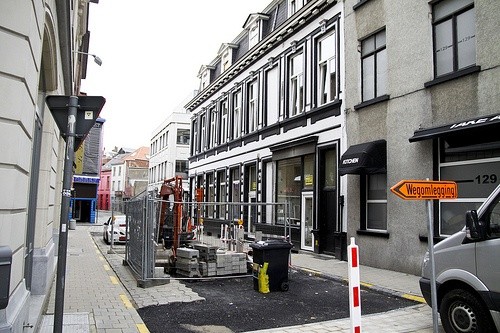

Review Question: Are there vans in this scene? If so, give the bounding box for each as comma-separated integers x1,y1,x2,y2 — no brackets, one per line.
418,184,500,333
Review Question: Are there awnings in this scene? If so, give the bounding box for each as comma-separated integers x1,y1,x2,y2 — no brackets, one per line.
338,139,387,178
409,114,500,143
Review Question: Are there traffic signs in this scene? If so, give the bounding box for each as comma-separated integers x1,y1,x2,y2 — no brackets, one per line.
390,181,459,202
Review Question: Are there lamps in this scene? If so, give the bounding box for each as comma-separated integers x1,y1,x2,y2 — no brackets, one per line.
71,50,102,66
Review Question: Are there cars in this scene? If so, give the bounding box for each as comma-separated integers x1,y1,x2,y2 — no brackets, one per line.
103,215,131,246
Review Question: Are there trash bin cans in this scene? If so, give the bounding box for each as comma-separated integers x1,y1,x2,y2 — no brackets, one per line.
247,236,294,294
